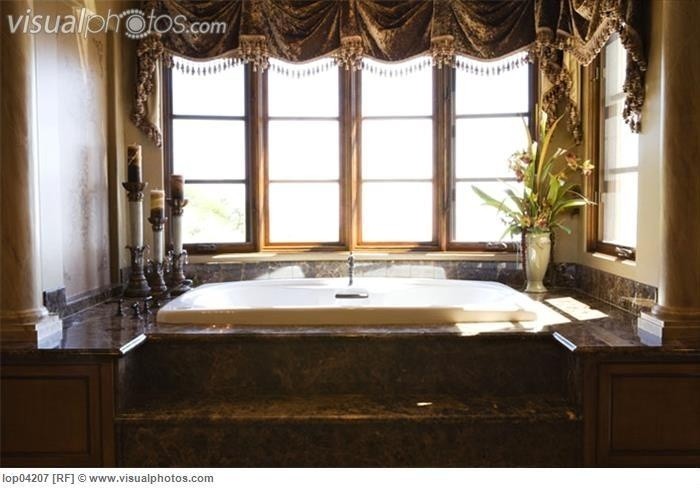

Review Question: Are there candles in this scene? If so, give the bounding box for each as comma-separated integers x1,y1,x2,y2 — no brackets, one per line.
171,175,183,200
127,145,142,183
151,189,164,218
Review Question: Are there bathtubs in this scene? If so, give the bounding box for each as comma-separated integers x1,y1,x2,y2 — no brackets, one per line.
155,279,543,325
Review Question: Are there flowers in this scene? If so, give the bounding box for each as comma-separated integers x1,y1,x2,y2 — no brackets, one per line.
472,112,598,232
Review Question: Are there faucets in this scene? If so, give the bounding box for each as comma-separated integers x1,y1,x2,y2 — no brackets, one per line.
347,250,354,287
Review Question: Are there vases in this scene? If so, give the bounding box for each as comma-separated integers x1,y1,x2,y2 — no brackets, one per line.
524,232,551,292
